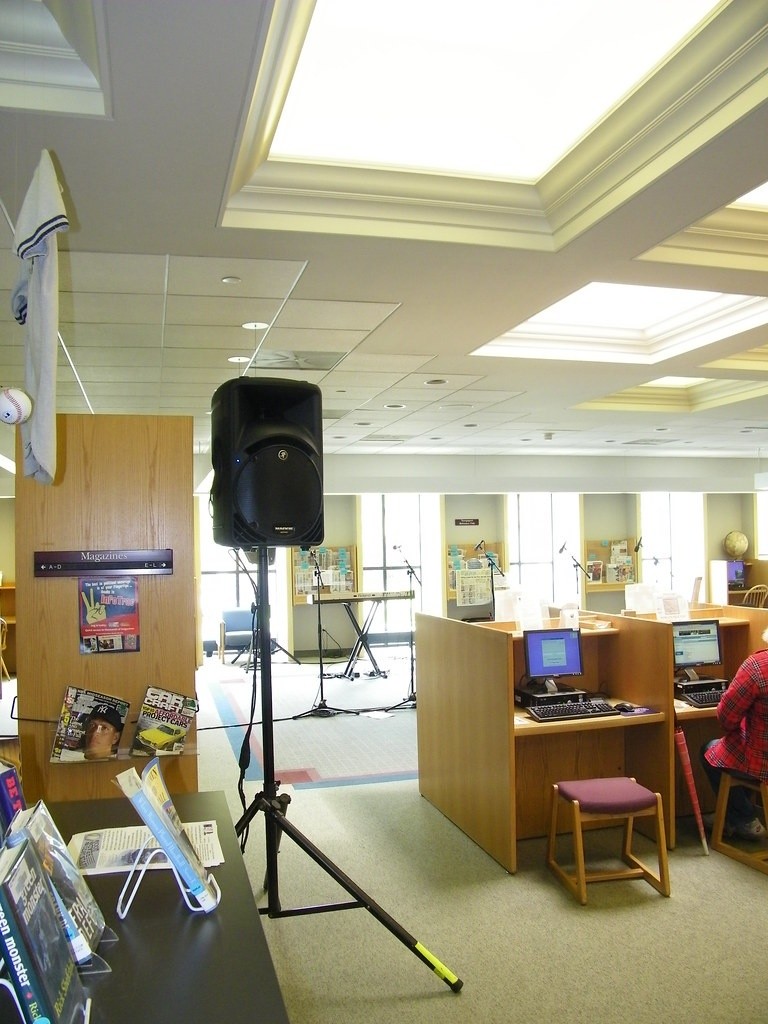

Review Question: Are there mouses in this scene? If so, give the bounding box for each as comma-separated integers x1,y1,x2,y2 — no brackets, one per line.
614,703,635,712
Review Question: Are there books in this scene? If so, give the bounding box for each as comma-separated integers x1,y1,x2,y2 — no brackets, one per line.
50,685,131,764
131,686,199,755
6,799,106,964
586,541,634,584
0,841,89,1023
112,756,217,913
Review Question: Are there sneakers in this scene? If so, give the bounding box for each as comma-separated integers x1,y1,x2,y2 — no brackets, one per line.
737,817,768,842
702,811,737,839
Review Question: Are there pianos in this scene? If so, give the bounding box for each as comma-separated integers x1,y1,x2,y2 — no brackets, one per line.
305,590,416,682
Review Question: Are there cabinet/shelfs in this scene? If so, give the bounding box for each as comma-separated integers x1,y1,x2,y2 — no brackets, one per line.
0,585,16,624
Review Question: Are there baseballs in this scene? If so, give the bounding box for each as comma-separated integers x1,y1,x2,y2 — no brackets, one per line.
0,388,32,424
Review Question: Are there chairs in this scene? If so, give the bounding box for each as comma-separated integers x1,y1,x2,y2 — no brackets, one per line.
0,618,10,699
740,585,768,608
217,609,259,664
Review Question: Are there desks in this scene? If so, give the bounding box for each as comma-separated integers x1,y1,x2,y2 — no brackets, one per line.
729,590,766,594
415,602,768,873
307,591,415,680
43,790,291,1024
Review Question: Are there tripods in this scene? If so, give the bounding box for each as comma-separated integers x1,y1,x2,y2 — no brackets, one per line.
231,545,464,992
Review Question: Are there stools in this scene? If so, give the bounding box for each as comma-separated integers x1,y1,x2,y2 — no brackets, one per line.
544,778,672,905
709,770,768,872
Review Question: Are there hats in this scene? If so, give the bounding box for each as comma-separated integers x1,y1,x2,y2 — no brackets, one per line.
77,705,122,731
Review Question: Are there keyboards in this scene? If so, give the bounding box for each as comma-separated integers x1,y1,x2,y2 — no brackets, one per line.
682,690,727,708
526,699,621,722
729,587,753,591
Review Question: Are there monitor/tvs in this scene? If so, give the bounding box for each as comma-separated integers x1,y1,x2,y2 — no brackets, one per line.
671,620,722,682
727,560,744,588
522,627,584,692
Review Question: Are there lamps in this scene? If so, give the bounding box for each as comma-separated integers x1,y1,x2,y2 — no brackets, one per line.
754,448,768,489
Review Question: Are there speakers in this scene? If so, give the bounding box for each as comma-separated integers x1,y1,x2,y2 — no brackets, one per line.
211,377,323,549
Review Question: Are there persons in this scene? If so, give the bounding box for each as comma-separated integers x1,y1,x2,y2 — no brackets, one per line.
698,623,768,838
75,706,119,758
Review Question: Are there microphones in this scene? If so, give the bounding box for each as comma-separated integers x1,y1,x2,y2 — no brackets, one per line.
474,540,483,550
393,546,401,549
559,543,566,553
310,549,316,556
634,537,643,552
231,547,240,550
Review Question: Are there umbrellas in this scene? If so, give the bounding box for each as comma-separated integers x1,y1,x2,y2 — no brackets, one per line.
674,709,710,856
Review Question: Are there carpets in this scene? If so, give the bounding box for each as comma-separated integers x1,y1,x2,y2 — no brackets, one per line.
207,664,420,791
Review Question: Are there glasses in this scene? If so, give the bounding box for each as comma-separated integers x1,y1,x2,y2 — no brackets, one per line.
87,723,115,735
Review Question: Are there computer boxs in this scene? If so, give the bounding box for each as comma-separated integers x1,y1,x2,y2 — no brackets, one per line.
513,688,589,710
674,678,729,700
